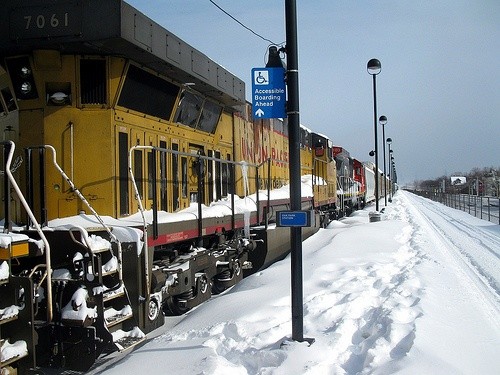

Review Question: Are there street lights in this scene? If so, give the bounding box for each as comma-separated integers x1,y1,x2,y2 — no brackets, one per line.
386,137,393,202
380,115,388,207
367,58,382,212
389,150,400,197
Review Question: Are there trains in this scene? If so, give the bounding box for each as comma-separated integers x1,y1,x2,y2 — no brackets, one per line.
0,0,398,374
